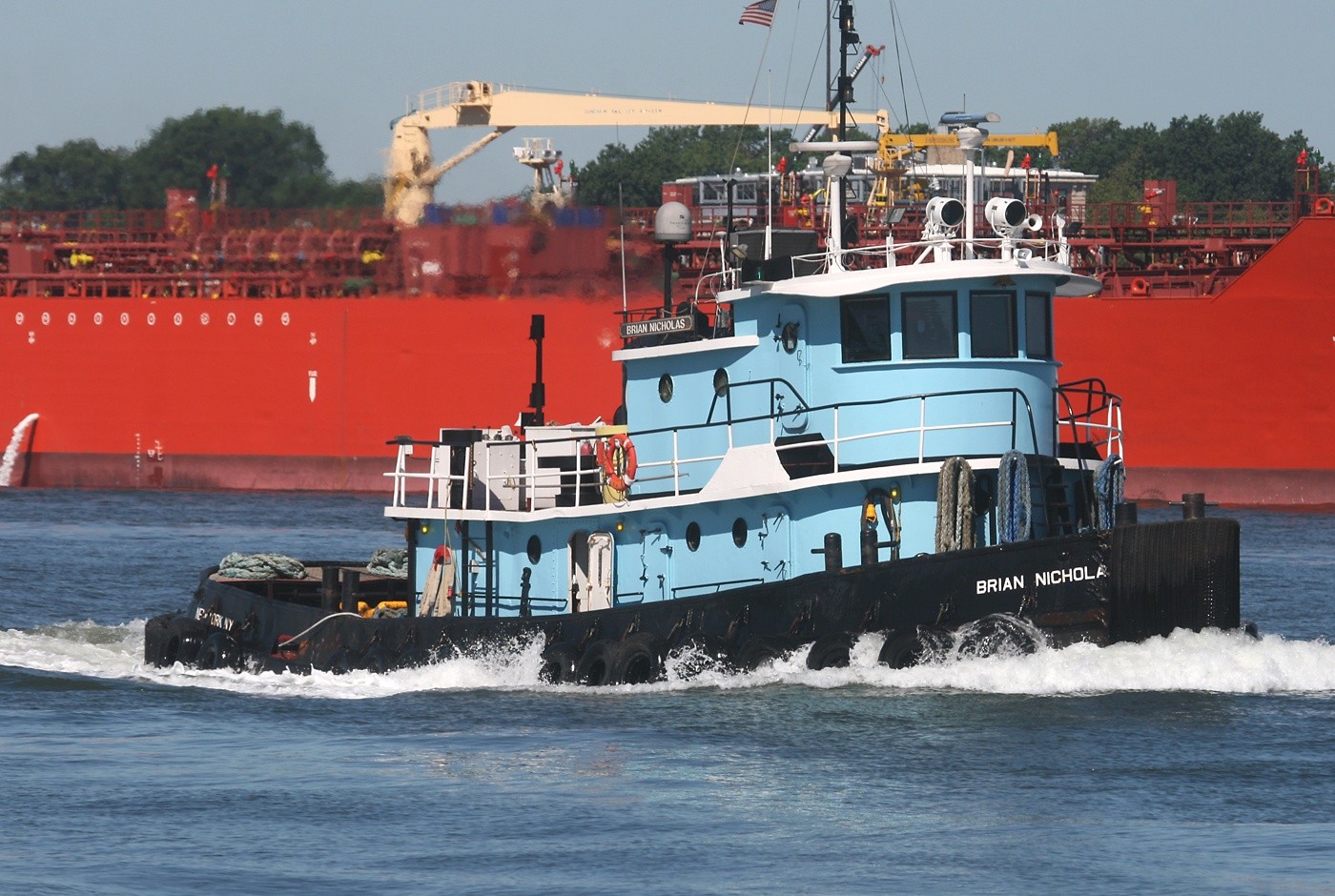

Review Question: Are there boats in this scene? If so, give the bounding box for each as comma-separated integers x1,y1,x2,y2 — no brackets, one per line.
145,0,1261,692
0,109,1334,585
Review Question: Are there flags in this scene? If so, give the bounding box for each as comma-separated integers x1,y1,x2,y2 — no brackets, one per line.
739,0,778,27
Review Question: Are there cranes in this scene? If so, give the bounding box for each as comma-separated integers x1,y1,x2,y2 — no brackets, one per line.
380,78,890,236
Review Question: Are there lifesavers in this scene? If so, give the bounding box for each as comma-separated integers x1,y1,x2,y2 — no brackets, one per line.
604,434,638,492
1314,197,1335,215
524,614,1049,686
155,617,239,673
1130,278,1147,294
431,544,456,601
